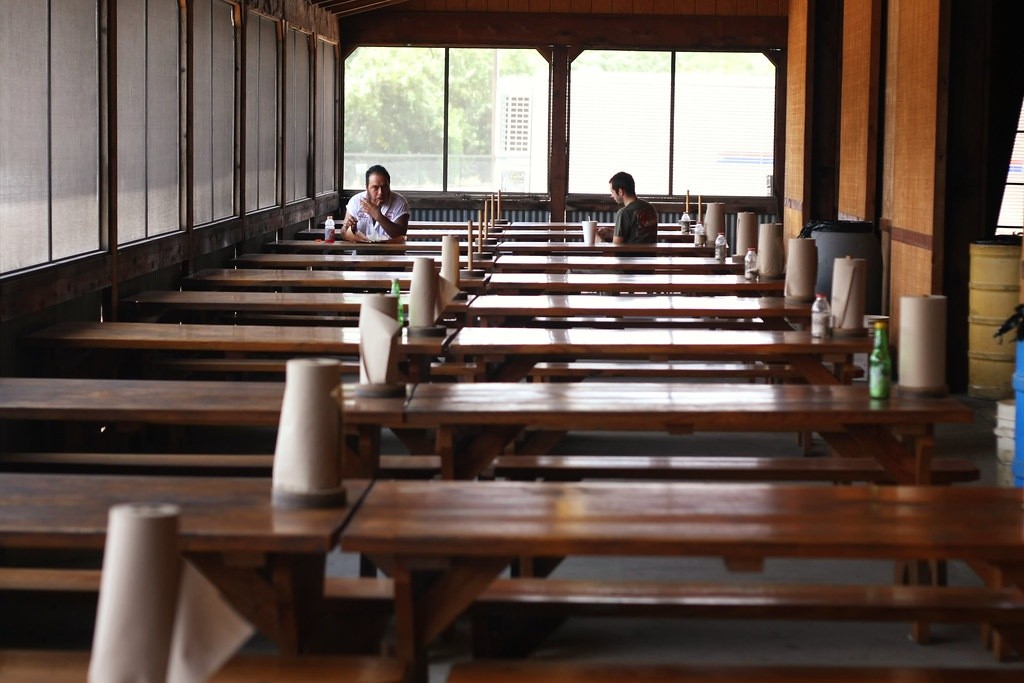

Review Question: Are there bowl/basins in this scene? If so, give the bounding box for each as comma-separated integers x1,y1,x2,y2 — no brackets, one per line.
863,315,890,328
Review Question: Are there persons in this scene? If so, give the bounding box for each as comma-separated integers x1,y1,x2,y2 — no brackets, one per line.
339,165,410,294
609,172,658,274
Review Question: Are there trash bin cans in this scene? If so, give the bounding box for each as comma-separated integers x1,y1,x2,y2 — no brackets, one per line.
1011,304,1024,488
806,219,874,316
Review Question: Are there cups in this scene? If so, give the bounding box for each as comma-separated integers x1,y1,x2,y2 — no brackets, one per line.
582,221,597,244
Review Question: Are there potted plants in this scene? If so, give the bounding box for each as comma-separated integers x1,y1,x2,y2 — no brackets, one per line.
993,303,1024,488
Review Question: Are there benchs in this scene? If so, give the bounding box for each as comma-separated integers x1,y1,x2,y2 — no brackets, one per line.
0,316,1024,683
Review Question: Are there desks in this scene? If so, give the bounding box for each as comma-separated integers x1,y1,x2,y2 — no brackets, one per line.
120,289,477,363
498,242,715,257
490,273,786,295
231,253,498,273
0,473,373,662
18,322,457,383
511,220,695,231
0,377,415,479
341,481,1024,683
265,240,501,255
178,268,490,296
503,230,695,243
402,384,973,644
468,295,830,384
495,255,746,275
447,327,895,481
334,220,511,231
297,228,507,243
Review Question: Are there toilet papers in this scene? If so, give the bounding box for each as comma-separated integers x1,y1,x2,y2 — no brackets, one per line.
828,256,868,330
783,236,818,298
269,355,343,490
407,256,461,329
358,291,404,388
704,200,726,240
87,501,257,682
735,211,757,257
756,222,783,278
897,293,948,389
441,232,460,292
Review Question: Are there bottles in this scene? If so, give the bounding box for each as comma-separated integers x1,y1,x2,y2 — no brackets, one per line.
695,221,705,246
745,248,759,280
681,212,690,233
390,278,404,326
325,216,335,243
810,294,831,338
715,233,727,260
868,323,891,398
